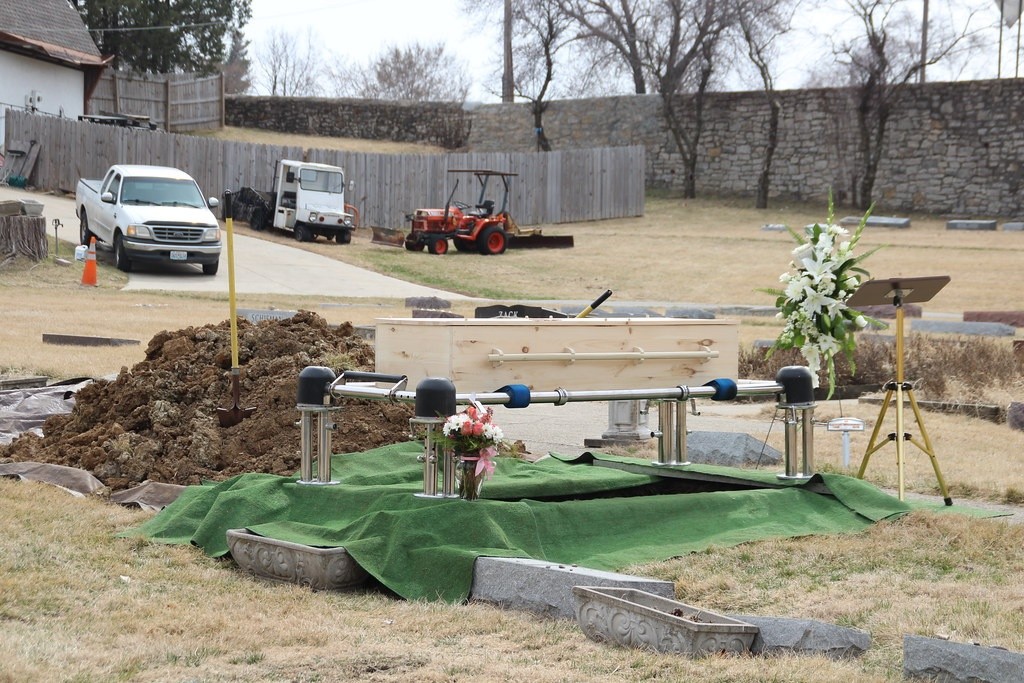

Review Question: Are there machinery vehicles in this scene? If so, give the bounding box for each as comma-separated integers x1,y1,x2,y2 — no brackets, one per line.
403,169,576,255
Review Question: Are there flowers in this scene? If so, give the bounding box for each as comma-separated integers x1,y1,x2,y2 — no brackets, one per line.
758,185,877,401
424,408,502,476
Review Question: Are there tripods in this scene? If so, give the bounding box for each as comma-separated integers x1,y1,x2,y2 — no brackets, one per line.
845,275,953,508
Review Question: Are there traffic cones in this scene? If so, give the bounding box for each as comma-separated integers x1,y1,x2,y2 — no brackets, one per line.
79,236,102,288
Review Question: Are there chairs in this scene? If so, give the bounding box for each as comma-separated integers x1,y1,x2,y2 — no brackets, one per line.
468,201,495,219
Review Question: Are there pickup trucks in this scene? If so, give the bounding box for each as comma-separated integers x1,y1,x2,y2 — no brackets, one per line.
75,164,223,275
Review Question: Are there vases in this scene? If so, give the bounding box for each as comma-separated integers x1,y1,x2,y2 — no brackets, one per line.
458,457,487,501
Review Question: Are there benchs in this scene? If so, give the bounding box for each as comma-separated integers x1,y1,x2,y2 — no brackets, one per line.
281,191,296,208
